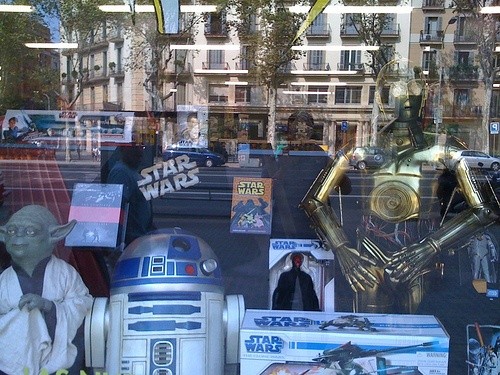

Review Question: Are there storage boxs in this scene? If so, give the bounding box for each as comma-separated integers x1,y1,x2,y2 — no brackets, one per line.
240,309,450,375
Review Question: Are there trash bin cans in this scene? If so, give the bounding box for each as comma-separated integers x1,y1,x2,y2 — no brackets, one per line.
98,144,154,237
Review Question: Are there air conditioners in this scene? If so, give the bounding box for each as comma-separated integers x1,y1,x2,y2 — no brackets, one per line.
422,34,432,41
355,63,363,71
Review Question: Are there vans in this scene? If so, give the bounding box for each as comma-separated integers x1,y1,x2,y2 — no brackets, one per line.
163,145,226,168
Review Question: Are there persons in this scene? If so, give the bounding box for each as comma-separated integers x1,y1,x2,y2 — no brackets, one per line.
272,254,321,312
302,59,500,315
262,110,353,238
468,231,496,284
3,116,28,140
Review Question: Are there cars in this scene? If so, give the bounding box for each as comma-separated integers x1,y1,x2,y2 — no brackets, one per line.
456,150,500,171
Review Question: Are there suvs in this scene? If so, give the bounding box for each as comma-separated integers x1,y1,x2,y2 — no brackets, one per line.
348,147,394,170
